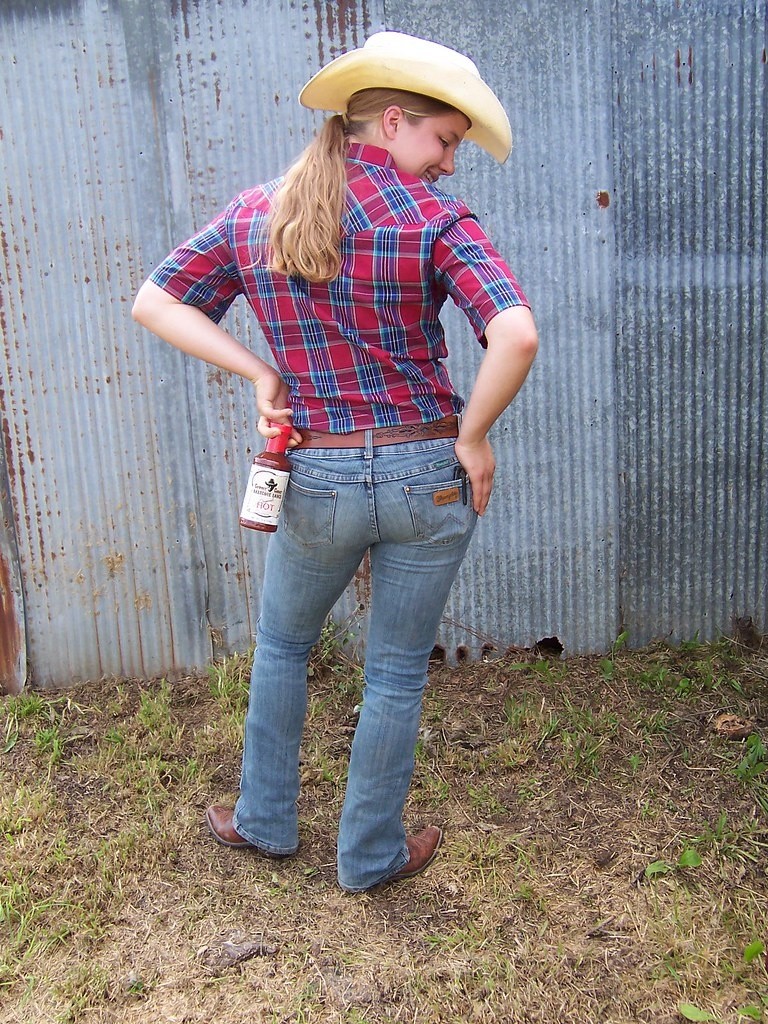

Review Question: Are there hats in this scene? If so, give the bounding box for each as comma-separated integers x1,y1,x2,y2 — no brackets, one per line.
300,31,513,165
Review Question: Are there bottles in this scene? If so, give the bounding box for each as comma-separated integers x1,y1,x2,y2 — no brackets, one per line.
238,422,292,534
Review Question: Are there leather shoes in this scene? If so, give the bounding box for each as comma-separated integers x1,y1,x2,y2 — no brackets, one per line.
207,805,288,860
339,826,443,891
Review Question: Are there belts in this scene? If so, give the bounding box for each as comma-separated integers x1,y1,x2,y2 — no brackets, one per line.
292,414,464,449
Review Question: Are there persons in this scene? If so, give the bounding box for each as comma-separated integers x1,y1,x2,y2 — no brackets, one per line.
133,31,539,891
